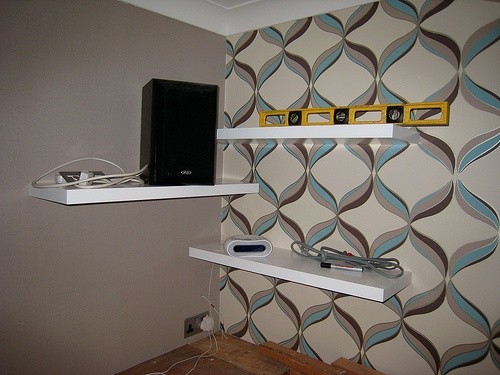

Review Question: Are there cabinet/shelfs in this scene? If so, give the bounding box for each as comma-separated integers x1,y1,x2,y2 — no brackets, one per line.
28,119,423,304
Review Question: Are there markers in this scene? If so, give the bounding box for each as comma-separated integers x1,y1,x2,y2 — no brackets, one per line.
320,262,363,273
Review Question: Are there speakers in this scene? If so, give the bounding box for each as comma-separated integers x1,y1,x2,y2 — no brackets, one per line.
140,76,218,187
